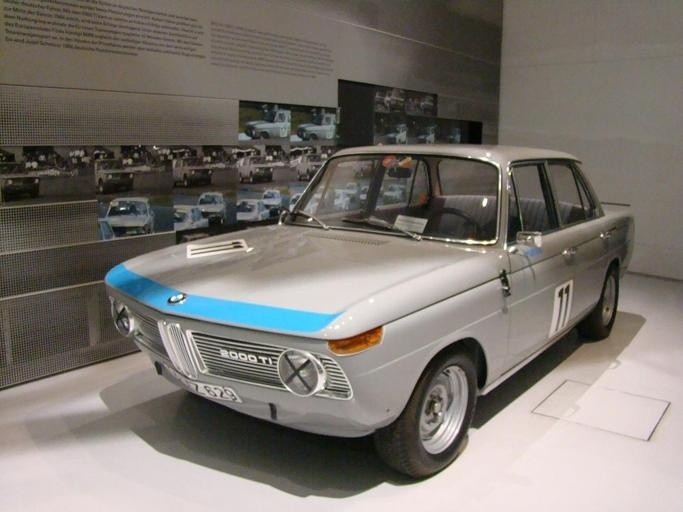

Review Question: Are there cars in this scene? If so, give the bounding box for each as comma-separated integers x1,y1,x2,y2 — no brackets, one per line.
103,143,635,477
245,90,462,145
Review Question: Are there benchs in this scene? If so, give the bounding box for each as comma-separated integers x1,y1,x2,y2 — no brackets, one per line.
425,195,588,241
